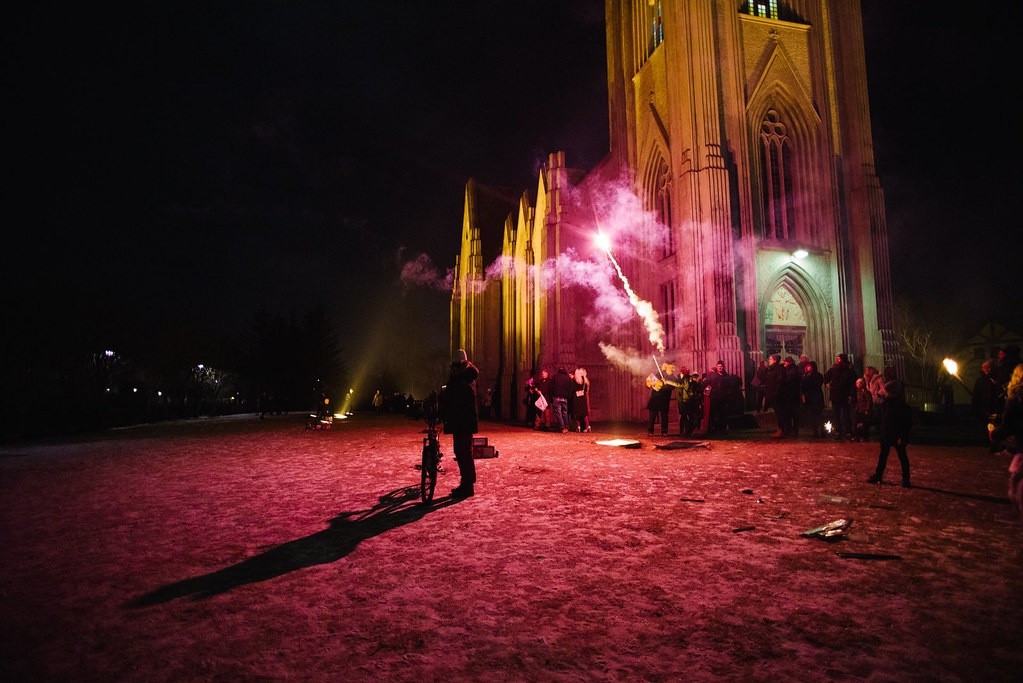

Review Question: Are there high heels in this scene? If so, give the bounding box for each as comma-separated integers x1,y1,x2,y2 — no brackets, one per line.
576,426,581,433
584,426,591,433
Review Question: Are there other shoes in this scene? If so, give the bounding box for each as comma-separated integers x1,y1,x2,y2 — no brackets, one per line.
867,475,883,484
544,426,552,431
835,429,880,442
663,433,667,436
535,426,541,430
562,428,568,433
706,428,713,436
811,430,819,437
681,432,693,438
650,432,653,436
450,478,474,498
820,429,827,437
995,449,1014,458
901,479,911,488
770,427,800,437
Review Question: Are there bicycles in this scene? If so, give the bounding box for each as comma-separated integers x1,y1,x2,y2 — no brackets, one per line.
415,410,446,506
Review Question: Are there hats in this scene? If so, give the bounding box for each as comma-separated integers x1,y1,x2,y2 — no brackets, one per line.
772,354,781,363
452,349,467,362
782,357,793,363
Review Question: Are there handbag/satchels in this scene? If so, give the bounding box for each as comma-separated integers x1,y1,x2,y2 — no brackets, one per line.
575,390,585,397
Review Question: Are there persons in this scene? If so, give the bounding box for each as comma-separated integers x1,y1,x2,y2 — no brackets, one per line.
968,347,1022,505
256,398,266,419
858,379,914,489
308,378,497,434
642,351,914,438
521,369,592,434
438,349,481,501
